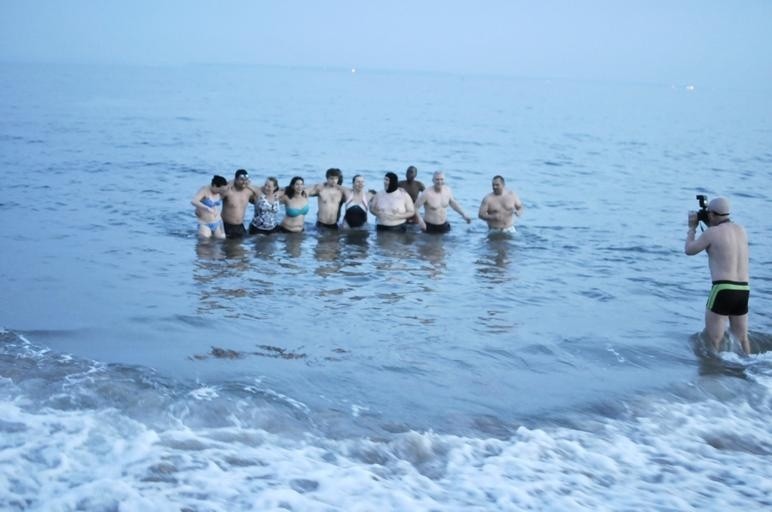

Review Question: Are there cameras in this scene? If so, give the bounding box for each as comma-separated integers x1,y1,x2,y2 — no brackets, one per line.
690,195,710,226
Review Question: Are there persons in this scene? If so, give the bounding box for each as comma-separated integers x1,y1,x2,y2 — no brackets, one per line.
246,177,286,235
332,174,376,231
278,175,320,235
476,174,524,235
369,172,416,234
413,170,474,234
396,165,427,225
189,175,228,241
684,195,755,365
308,167,344,230
218,168,252,240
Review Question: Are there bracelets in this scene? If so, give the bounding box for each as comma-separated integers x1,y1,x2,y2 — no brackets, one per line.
687,228,696,234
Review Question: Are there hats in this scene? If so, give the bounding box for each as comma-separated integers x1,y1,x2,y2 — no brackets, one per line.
706,196,732,215
383,172,399,193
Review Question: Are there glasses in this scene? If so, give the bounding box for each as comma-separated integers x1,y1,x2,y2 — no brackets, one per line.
239,173,249,179
